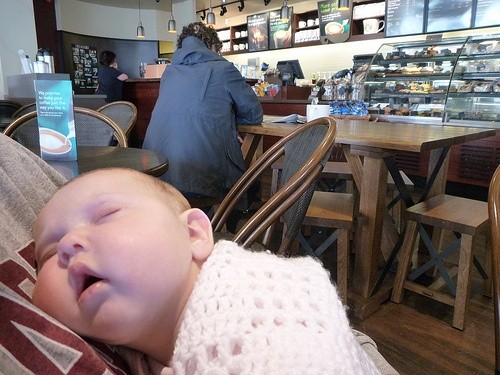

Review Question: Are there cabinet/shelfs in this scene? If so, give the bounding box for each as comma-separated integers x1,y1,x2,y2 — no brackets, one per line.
363,33,500,187
292,9,321,48
215,23,249,55
350,0,385,42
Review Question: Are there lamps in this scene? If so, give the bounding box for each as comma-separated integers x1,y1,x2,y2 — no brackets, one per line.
219,5,227,16
280,0,289,20
264,0,272,6
337,0,349,10
200,10,206,21
168,0,177,32
238,0,245,12
207,0,216,25
137,0,145,37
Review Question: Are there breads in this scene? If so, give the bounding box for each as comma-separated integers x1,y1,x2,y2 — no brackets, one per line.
381,66,445,117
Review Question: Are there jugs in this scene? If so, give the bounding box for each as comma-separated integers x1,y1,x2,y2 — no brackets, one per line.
33,48,55,74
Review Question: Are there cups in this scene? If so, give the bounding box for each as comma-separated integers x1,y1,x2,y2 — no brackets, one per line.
363,18,386,35
233,30,248,50
299,18,319,28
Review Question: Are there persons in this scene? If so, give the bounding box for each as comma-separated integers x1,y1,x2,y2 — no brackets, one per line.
0,130,400,375
31,167,381,375
95,50,128,104
142,22,263,235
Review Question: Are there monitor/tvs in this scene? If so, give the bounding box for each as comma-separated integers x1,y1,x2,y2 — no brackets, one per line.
278,59,305,79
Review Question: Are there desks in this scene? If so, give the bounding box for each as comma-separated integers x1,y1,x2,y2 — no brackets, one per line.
239,119,496,319
28,146,169,180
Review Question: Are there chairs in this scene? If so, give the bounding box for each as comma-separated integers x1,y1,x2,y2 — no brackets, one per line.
0,100,500,375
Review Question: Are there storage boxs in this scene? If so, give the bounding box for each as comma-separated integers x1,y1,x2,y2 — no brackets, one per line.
6,73,70,97
143,64,167,79
